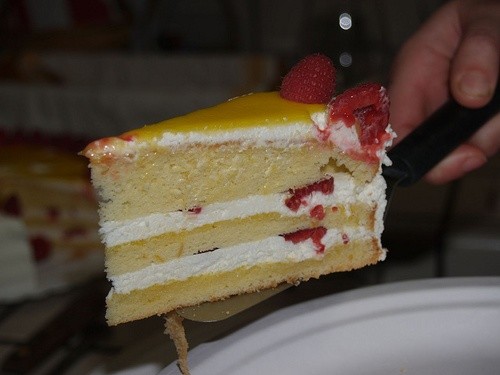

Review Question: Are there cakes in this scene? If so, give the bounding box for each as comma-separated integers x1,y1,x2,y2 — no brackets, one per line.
77,53,396,326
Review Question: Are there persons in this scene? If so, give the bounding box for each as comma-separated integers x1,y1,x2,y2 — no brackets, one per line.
386,0,500,185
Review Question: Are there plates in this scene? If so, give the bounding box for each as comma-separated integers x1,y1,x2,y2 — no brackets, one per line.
158,275,500,375
